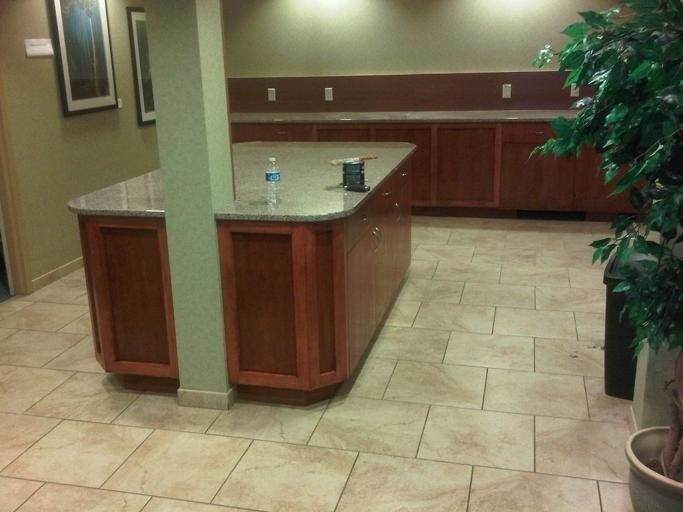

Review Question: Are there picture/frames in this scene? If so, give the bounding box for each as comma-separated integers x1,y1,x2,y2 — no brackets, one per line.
49,0,119,116
126,6,157,126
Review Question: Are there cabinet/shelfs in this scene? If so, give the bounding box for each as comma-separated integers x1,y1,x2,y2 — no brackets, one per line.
499,122,655,219
434,121,499,208
347,155,411,385
306,218,347,405
216,220,306,406
314,121,434,208
77,214,178,394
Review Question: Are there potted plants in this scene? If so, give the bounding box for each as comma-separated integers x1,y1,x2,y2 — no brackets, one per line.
529,0,683,512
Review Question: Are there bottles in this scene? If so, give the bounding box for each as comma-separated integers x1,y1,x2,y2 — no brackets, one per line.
342,160,365,189
265,157,280,206
